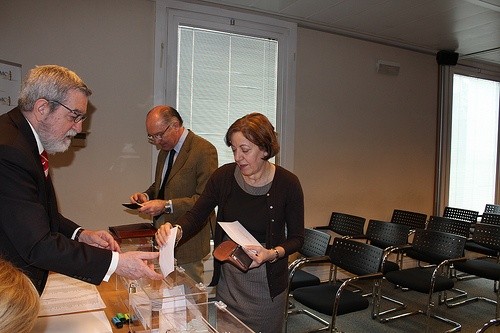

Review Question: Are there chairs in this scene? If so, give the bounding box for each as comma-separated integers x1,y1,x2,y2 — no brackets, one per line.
283,204,500,333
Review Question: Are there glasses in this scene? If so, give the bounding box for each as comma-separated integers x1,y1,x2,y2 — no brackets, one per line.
47,99,88,124
148,125,170,140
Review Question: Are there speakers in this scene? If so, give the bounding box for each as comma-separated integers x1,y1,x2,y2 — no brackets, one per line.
436,51,459,66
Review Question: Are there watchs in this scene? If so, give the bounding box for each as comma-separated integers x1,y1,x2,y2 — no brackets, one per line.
163,201,171,214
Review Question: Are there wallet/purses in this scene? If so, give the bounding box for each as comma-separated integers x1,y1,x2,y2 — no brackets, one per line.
212,241,253,273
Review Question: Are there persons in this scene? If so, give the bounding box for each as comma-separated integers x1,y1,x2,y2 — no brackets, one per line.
0,64,165,298
130,106,219,284
154,113,305,333
0,259,40,333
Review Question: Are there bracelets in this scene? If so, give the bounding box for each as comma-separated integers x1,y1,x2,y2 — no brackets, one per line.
269,248,279,264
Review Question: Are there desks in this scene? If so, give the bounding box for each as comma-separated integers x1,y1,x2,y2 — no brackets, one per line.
33,244,255,333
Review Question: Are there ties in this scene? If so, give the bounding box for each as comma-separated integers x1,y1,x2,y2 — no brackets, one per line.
154,149,176,200
40,149,49,180
